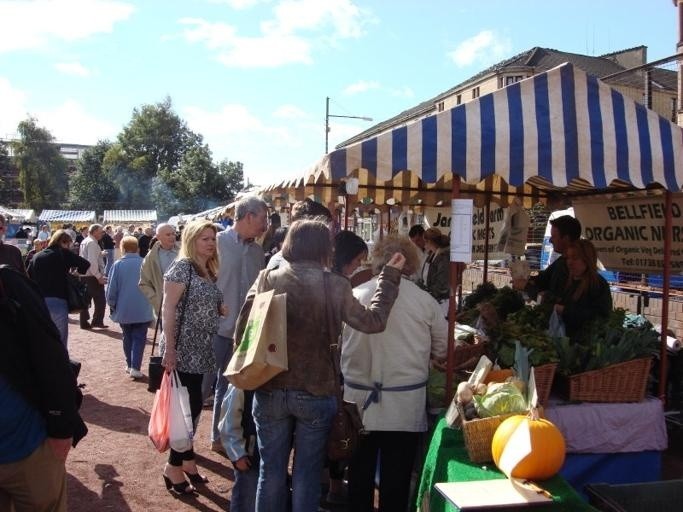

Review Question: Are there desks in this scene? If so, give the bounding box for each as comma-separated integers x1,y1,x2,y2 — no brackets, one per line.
411,415,598,512
543,396,669,502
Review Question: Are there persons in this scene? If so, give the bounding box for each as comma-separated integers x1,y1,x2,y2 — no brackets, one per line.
510,215,582,302
78,224,180,379
157,198,451,512
555,238,612,330
1,212,90,512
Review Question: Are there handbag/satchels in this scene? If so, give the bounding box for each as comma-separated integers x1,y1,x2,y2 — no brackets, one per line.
148,356,162,392
329,398,364,459
223,290,289,392
66,270,90,314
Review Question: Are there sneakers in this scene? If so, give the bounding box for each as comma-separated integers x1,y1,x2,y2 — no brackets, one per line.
202,394,215,407
125,365,144,378
321,480,348,504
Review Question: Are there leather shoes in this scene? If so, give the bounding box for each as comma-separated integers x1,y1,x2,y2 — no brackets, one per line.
80,323,109,328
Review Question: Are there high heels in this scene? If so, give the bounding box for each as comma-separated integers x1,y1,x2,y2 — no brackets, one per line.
163,474,194,494
184,469,208,484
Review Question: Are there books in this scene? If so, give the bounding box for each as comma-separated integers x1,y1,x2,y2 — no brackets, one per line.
433,478,555,511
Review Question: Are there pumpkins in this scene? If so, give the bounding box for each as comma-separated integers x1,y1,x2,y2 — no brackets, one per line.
491,407,566,481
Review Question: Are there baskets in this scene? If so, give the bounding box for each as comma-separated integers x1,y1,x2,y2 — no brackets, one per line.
463,361,558,408
455,402,544,463
568,356,654,402
